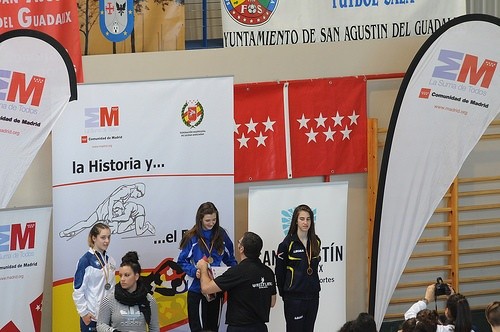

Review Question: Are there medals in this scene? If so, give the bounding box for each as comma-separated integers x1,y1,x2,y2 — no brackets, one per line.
207,257,213,263
307,268,313,275
105,283,111,290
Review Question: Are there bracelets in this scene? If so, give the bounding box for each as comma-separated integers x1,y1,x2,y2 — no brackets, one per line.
421,298,428,305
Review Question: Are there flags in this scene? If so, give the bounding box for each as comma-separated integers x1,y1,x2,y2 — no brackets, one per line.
368,13,500,332
0,28,78,209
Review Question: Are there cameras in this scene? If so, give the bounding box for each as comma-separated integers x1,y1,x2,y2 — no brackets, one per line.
435,283,451,296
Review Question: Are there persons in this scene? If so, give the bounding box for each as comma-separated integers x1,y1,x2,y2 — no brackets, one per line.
275,205,321,332
396,283,475,332
72,223,116,332
96,250,160,332
485,300,500,332
197,232,277,332
177,202,238,332
337,312,378,332
